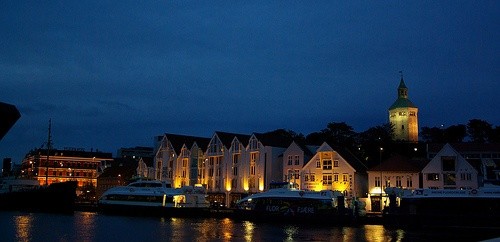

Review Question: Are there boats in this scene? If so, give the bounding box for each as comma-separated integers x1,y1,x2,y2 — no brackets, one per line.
98,176,211,215
381,185,499,231
234,180,347,226
